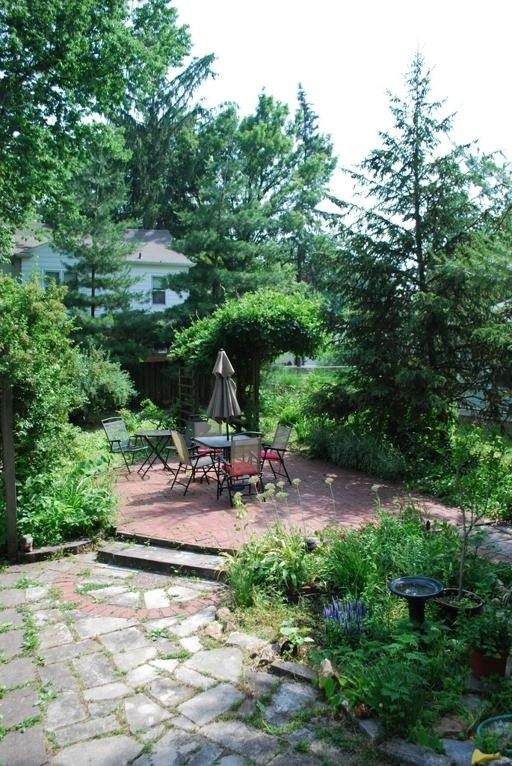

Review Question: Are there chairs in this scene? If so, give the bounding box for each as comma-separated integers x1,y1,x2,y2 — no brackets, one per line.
256,423,294,486
171,430,223,497
190,422,222,483
161,429,201,473
102,416,154,474
222,431,268,508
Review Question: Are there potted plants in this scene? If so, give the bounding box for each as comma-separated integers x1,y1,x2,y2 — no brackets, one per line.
410,418,512,632
470,713,511,758
455,604,511,681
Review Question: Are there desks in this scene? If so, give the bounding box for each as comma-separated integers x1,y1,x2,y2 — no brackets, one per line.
195,435,252,500
134,429,179,479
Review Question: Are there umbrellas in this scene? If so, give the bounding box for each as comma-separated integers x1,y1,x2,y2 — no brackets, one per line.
205,346,244,440
